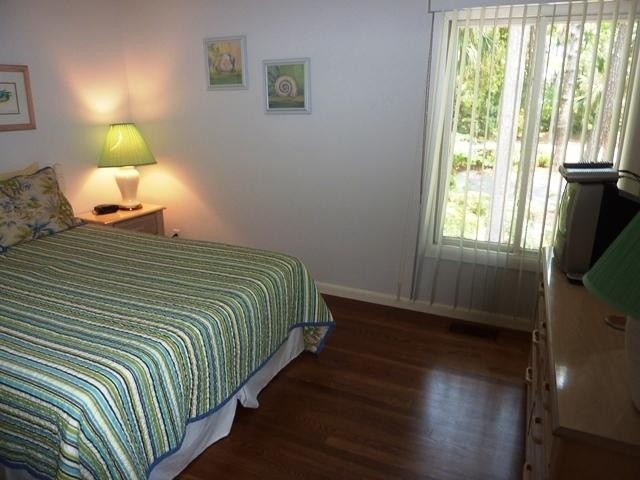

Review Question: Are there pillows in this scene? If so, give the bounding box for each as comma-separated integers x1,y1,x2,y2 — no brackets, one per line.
0,160,40,185
0,166,88,261
47,161,69,199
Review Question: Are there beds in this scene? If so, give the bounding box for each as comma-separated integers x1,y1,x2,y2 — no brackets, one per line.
0,216,338,480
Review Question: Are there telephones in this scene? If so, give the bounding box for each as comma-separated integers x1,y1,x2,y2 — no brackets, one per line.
94,204,119,214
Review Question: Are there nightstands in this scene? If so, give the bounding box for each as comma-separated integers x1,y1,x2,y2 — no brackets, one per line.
73,199,169,240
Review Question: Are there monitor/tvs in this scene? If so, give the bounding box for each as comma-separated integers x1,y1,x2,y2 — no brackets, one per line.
554,182,640,273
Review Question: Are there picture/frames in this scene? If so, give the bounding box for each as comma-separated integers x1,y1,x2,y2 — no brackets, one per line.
0,62,39,133
262,56,314,118
202,32,251,94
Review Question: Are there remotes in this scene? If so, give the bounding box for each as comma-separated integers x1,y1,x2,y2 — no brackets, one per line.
567,273,583,285
564,161,613,168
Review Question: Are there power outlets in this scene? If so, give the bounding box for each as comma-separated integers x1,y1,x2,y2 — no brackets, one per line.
173,228,182,239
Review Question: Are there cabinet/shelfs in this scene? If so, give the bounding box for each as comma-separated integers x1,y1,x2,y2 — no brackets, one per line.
518,245,638,480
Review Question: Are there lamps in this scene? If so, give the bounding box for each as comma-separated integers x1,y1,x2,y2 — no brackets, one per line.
94,121,160,211
578,205,639,421
